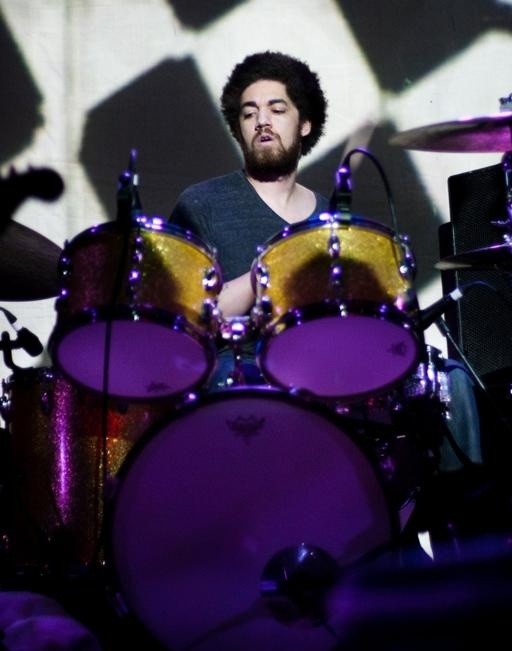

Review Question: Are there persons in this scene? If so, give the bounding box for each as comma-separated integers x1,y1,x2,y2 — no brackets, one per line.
168,53,333,316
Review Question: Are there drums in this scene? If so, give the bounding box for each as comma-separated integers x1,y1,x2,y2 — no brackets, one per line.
48,216,223,406
251,211,425,406
1,366,167,581
101,383,402,650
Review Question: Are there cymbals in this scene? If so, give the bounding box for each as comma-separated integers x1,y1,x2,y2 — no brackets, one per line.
0,219,62,300
386,108,512,152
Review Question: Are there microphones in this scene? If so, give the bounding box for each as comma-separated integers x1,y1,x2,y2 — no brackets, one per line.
408,289,467,334
5,309,45,357
